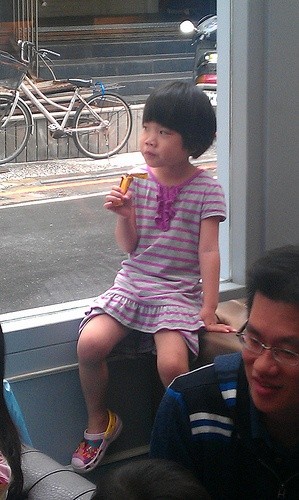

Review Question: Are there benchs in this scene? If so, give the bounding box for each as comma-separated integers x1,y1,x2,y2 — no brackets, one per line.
199,295,247,420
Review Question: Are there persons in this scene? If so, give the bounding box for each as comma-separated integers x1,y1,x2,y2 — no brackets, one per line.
89,457,213,500
147,244,299,500
71,80,238,475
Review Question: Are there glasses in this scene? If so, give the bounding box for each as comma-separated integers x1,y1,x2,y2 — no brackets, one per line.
236,319,299,366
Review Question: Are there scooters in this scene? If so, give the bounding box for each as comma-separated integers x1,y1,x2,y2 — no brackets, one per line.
180,11,217,108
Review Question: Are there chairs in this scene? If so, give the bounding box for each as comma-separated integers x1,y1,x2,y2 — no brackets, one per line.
21,443,96,500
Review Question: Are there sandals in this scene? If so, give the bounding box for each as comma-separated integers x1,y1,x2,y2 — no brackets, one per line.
71,408,123,474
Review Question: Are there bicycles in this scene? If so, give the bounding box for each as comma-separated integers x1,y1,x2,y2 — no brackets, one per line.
0,39,134,165
